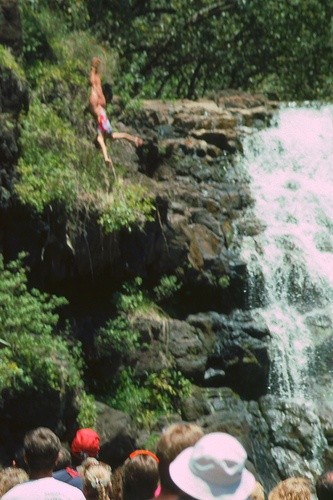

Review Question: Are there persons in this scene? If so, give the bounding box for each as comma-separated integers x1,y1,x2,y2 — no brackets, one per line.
89,57,144,167
0,423,333,500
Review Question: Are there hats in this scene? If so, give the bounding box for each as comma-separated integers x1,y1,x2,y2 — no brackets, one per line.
70,427,100,460
168,433,256,500
